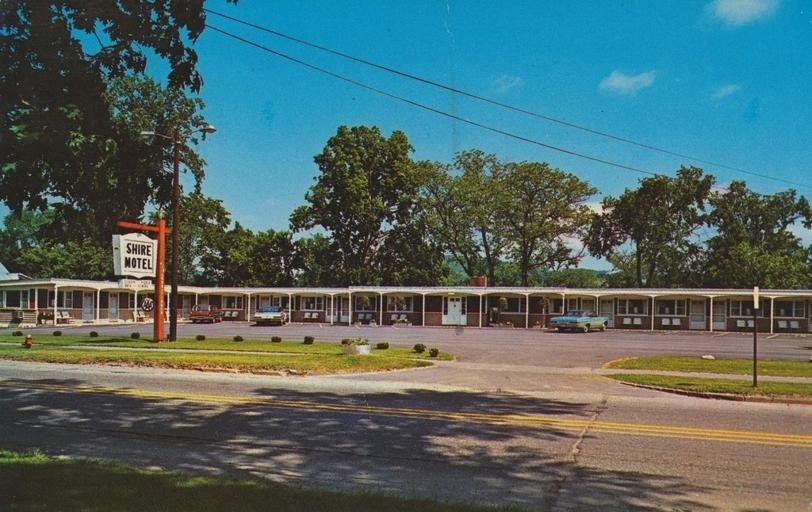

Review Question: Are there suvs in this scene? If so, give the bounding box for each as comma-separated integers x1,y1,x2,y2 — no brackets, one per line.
190,304,224,322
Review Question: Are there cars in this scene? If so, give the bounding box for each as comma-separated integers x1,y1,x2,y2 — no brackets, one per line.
253,306,287,326
548,309,609,333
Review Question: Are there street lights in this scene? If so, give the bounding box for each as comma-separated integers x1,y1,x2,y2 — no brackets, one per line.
138,124,217,343
